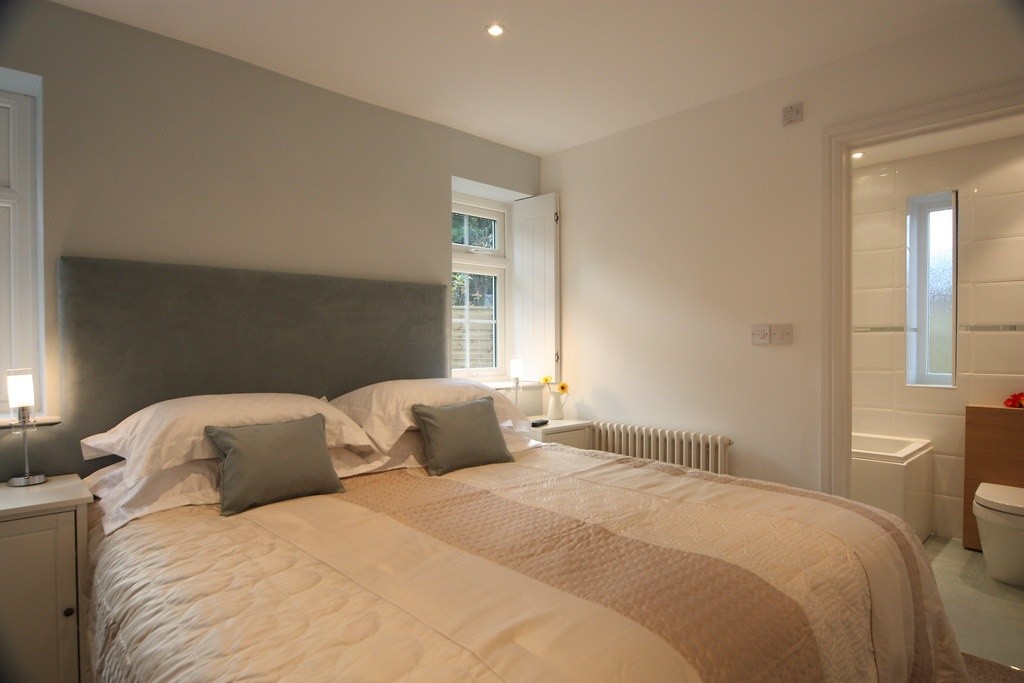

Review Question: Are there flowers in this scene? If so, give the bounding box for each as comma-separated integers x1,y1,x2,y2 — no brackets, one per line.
1004,392,1024,409
539,377,571,395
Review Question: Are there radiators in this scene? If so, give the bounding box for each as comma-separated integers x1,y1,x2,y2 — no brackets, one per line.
588,422,733,475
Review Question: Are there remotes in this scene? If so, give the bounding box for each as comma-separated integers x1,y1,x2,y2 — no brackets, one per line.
531,419,549,427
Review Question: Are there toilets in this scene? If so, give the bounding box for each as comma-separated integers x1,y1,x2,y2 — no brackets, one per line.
971,480,1024,588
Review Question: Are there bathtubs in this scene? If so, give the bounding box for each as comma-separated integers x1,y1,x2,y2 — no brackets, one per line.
850,431,936,544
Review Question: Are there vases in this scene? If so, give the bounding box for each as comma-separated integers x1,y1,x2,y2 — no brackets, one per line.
548,393,566,420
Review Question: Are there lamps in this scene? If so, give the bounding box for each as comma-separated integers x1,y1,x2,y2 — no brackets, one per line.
7,369,48,487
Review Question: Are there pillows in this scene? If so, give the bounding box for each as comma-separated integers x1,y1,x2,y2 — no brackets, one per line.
332,376,528,437
84,457,377,535
358,433,543,473
75,392,378,469
206,414,346,516
410,398,517,474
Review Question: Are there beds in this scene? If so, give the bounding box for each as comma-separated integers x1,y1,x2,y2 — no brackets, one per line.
56,256,970,683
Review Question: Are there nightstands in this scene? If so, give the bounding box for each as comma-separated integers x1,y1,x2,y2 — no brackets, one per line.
0,473,94,683
524,415,587,447
